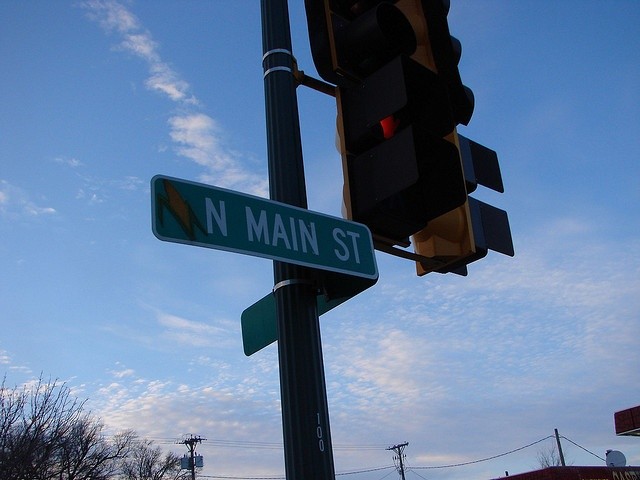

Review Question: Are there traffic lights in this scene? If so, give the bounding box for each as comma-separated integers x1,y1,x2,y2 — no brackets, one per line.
326,0,466,244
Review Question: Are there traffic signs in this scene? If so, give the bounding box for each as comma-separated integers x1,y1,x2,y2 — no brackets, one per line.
150,174,378,280
